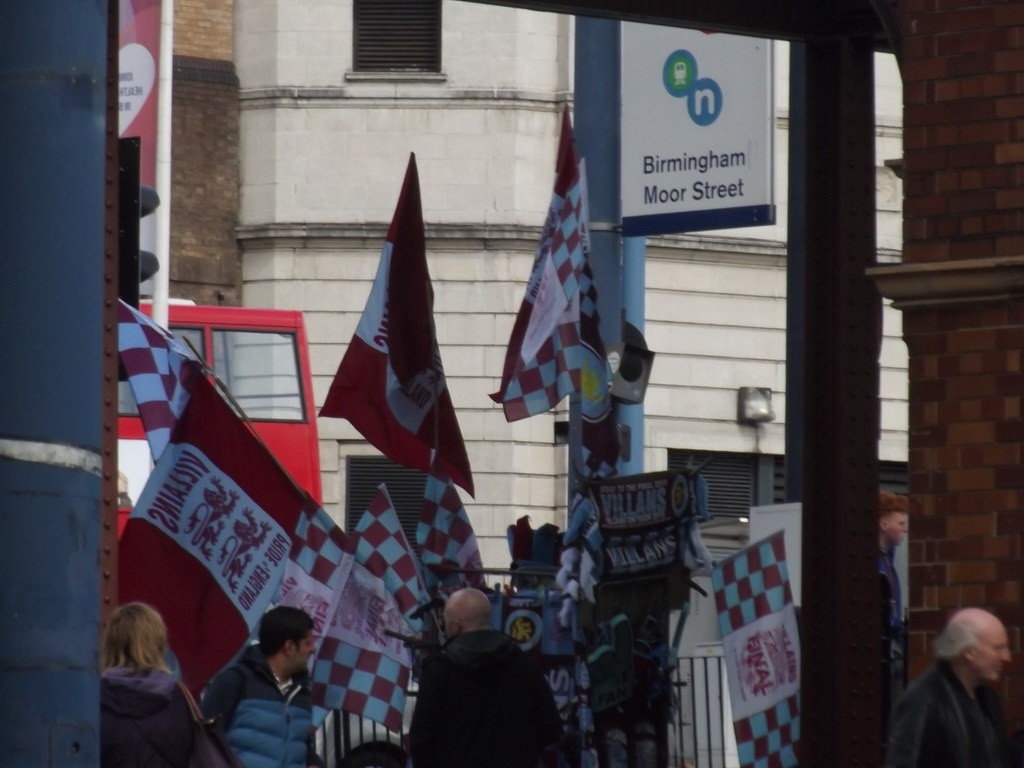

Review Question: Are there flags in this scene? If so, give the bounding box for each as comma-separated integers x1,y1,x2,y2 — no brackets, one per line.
490,108,583,422
278,509,348,736
307,490,425,733
318,156,476,500
116,361,306,705
117,298,191,465
710,530,801,768
577,263,623,471
412,474,486,684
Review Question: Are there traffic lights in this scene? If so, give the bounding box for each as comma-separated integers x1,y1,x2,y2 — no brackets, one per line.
117,137,160,380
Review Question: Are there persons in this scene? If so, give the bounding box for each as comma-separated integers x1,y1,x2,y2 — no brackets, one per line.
409,588,564,768
883,607,1012,768
878,491,909,748
202,606,323,768
100,601,195,768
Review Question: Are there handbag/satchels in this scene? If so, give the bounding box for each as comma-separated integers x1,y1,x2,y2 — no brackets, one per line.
176,680,247,768
